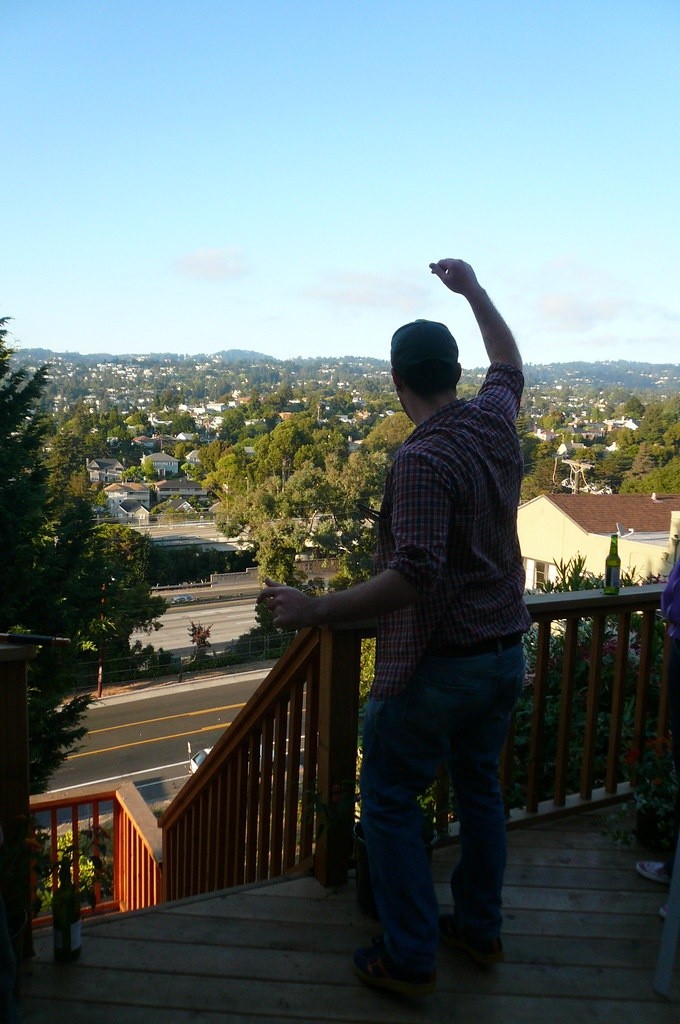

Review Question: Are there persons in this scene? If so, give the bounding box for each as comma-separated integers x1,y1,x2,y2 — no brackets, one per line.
634,551,680,920
254,257,531,999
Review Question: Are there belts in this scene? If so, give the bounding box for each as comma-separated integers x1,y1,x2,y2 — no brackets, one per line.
421,631,522,658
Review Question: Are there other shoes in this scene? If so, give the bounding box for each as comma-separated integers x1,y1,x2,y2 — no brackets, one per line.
636,860,671,883
658,904,670,919
351,934,435,994
436,913,504,963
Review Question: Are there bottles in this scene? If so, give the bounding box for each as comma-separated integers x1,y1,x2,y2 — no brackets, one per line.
53,857,81,962
603,534,620,595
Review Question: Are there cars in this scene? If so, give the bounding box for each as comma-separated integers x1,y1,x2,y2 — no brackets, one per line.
188,746,282,787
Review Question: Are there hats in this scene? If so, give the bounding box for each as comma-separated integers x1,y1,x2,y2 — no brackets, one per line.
390,319,458,380
